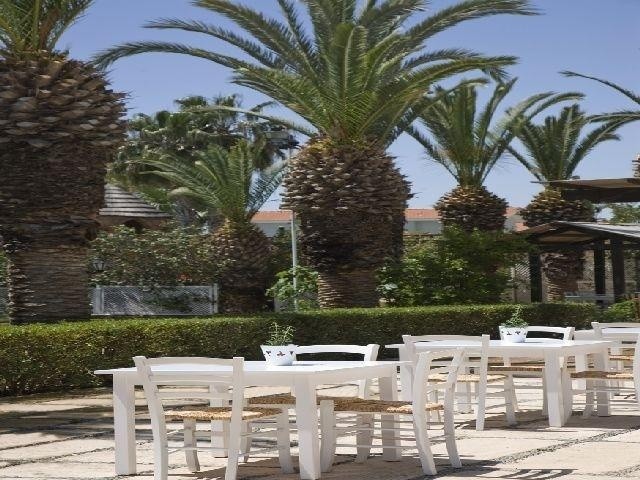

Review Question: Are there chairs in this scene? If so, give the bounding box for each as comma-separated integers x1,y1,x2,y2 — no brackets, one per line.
473,324,575,416
571,321,639,419
241,342,380,471
316,347,466,476
401,333,516,432
133,354,294,479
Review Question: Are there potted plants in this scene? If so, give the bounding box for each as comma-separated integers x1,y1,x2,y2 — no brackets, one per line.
499,303,529,343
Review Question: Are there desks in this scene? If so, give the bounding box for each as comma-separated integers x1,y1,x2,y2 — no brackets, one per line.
93,360,414,477
573,326,639,396
384,340,616,428
